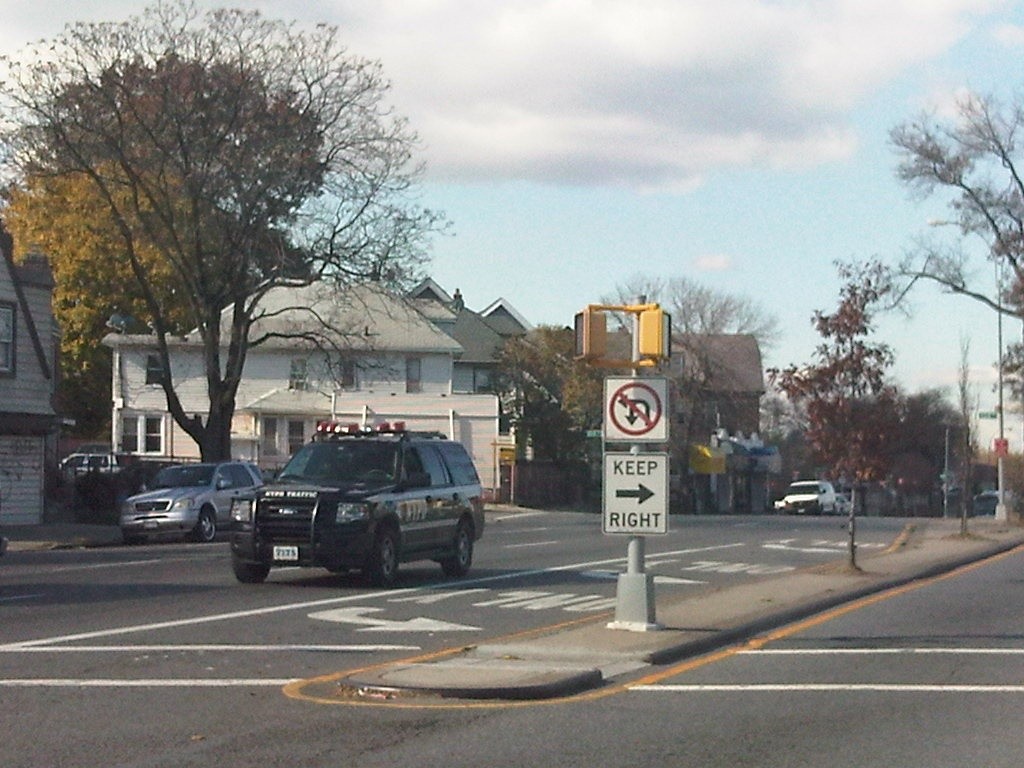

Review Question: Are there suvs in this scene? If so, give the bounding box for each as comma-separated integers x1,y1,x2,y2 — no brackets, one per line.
773,479,863,518
227,420,488,590
118,458,270,546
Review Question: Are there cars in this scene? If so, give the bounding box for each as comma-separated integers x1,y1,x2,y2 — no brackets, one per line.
55,443,191,504
973,490,998,517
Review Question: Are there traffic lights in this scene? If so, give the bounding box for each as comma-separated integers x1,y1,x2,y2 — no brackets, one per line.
638,307,672,362
572,307,608,362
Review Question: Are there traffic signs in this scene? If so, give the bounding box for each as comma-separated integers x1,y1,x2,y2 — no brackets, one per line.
601,450,669,537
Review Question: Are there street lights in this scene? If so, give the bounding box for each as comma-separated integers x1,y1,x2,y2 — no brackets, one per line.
927,217,1007,519
